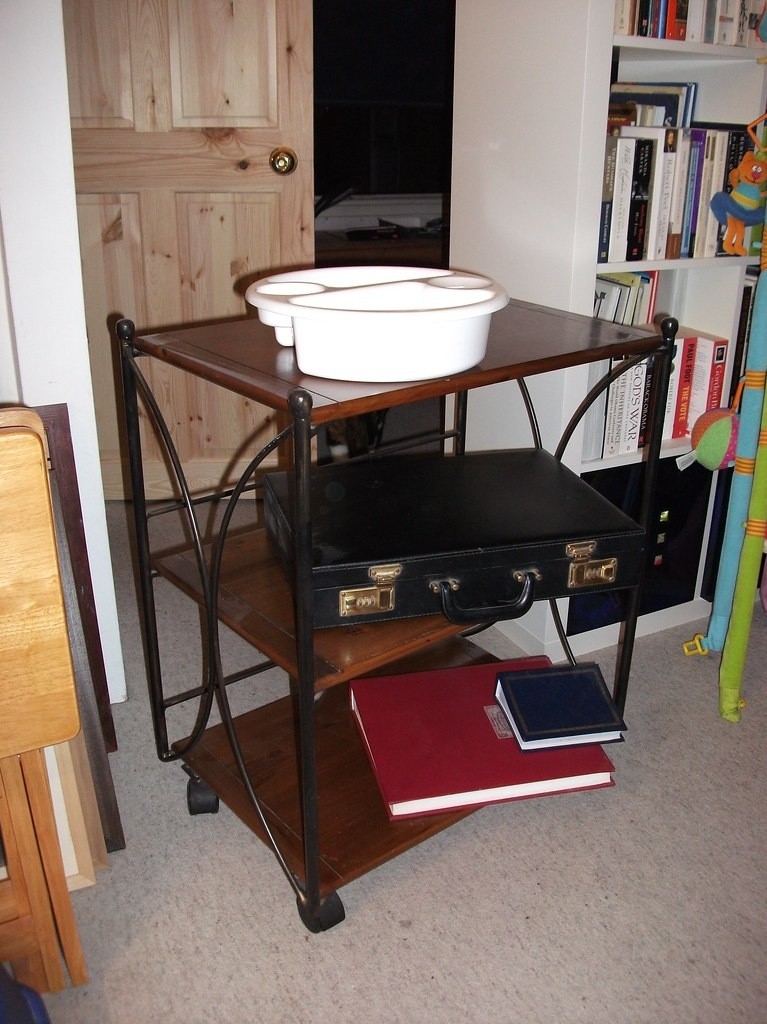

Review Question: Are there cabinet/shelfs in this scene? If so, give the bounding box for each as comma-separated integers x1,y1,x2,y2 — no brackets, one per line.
116,299,679,934
443,0,767,662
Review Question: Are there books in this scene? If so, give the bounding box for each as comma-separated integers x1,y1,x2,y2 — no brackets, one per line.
582,270,729,462
597,80,766,264
348,654,629,823
612,0,766,48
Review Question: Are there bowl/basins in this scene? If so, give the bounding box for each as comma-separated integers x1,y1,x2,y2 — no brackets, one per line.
245,265,511,383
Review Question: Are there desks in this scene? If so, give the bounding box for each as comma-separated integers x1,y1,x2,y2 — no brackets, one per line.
314,229,443,444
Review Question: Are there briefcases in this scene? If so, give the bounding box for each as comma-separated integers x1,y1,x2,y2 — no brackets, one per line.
262,448,644,630
